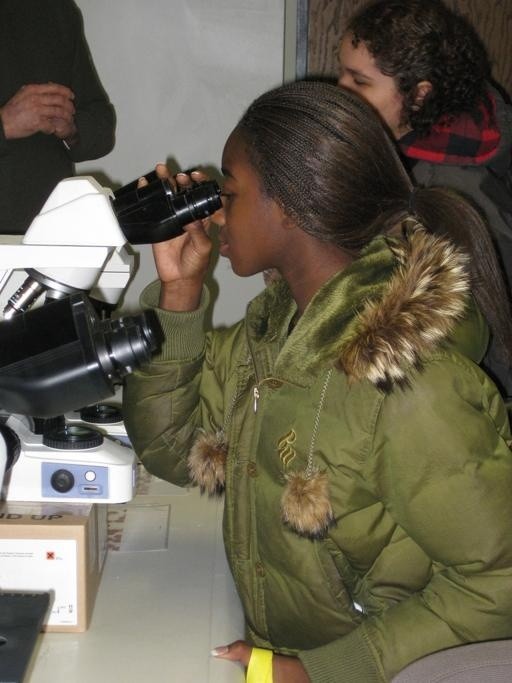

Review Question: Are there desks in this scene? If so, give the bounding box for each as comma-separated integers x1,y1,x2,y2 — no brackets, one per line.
21,463,246,683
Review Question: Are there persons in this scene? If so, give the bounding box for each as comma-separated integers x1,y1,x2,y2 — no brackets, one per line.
121,72,510,681
336,0,510,415
0,2,119,239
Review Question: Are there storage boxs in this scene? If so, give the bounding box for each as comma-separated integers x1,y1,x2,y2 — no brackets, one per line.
0,501,109,632
